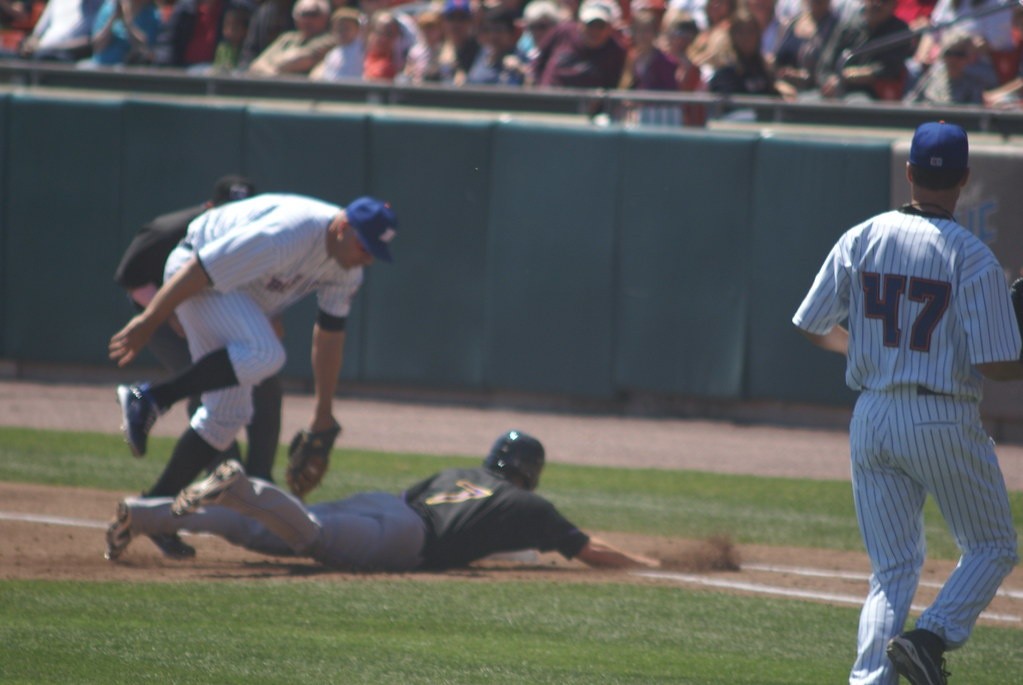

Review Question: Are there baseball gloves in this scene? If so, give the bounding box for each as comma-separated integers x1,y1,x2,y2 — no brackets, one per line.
286,424,341,496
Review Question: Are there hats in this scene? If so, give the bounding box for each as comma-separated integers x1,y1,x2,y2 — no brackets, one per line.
212,174,254,207
908,121,970,179
347,196,396,265
522,1,616,26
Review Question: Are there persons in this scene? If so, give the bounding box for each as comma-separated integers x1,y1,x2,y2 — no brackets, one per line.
108,431,661,572
794,120,1023,685
108,193,397,560
0,0,1023,135
116,177,285,484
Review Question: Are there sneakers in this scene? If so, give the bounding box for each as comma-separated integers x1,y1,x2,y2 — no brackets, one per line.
885,627,952,685
171,460,249,518
150,532,197,561
117,382,169,460
102,495,140,561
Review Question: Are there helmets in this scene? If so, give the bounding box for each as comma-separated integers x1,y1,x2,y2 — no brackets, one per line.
483,430,546,492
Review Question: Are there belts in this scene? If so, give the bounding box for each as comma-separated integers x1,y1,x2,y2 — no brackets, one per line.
861,383,953,398
176,238,192,252
